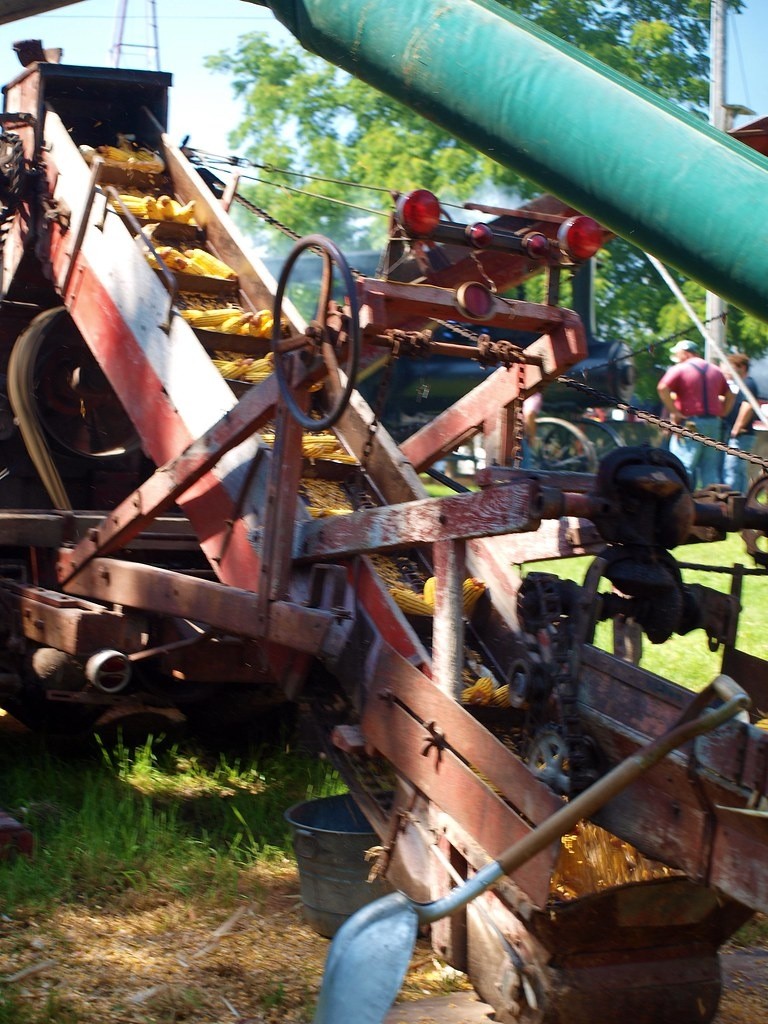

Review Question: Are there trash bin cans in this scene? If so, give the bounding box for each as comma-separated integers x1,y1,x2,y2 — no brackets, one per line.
283,792,399,941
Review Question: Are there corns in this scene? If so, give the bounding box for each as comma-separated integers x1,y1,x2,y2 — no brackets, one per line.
387,576,485,616
75,140,297,381
551,808,688,902
260,430,360,517
460,675,519,709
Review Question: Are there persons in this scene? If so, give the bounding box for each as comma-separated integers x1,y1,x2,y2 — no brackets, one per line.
658,385,671,450
657,340,730,489
722,353,758,492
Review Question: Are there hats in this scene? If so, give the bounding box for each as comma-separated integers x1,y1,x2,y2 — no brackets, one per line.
669,340,699,353
669,355,681,363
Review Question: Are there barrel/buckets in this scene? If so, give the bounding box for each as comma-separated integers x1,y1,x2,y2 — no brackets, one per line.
288,792,392,938
